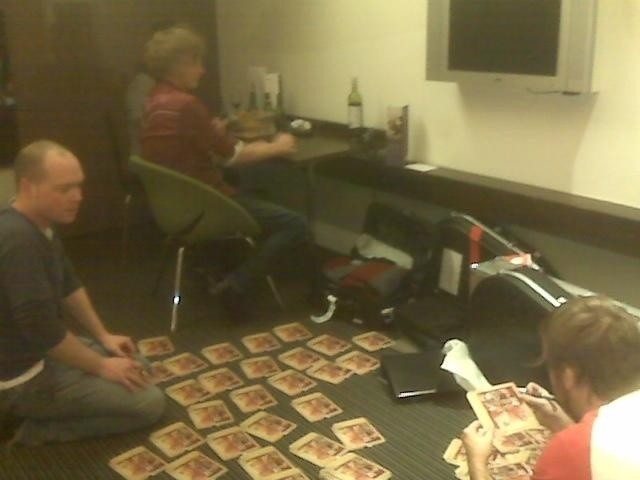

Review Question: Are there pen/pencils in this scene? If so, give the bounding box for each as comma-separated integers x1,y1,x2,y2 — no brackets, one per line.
519,391,556,399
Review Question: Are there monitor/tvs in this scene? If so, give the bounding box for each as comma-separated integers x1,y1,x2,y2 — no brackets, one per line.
425,0,591,95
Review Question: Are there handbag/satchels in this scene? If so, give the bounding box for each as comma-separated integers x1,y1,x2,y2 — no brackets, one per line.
311,258,401,328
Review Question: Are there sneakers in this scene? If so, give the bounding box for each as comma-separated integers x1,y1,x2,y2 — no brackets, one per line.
1,418,26,446
222,287,250,322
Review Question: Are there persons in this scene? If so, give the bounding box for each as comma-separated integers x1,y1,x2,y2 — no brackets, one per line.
139,26,358,317
0,138,166,452
125,17,175,158
459,294,640,480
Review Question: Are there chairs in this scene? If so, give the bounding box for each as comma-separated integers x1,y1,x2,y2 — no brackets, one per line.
102,107,149,265
126,155,292,333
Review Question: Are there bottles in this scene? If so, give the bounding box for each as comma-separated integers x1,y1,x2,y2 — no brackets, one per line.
247,91,282,110
348,76,363,138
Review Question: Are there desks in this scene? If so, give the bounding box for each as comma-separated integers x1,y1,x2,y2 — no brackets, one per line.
228,113,385,307
